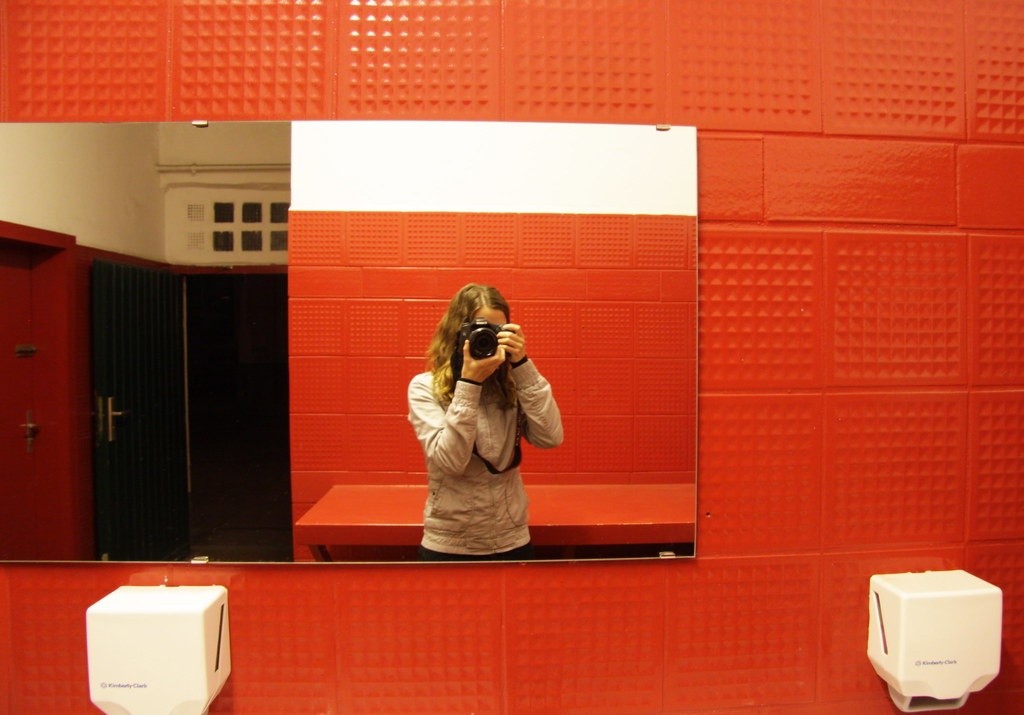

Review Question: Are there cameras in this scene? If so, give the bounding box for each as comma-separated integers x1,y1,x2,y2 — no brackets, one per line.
458,318,515,359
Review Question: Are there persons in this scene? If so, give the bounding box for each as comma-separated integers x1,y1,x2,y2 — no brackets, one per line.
407,283,564,561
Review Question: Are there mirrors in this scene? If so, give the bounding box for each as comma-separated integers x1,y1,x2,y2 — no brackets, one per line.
0,120,700,565
